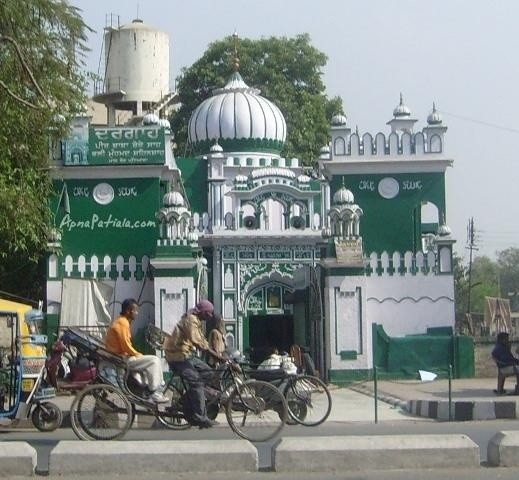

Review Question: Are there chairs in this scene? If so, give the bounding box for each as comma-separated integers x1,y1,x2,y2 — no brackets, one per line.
490,353,519,395
290,346,303,375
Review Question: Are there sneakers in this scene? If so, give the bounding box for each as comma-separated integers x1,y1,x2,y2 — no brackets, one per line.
142,390,169,402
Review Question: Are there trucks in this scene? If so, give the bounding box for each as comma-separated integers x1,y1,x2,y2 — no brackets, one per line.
0,298,49,390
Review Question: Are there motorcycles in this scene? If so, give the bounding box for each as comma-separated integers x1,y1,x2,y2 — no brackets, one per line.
214,342,307,427
44,332,97,391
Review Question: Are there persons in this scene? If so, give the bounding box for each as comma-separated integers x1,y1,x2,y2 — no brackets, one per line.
106,299,170,403
492,332,519,374
205,313,225,367
164,300,222,425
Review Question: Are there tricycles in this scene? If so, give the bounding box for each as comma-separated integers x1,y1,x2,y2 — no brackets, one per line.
0,297,65,433
63,319,291,441
147,325,335,430
